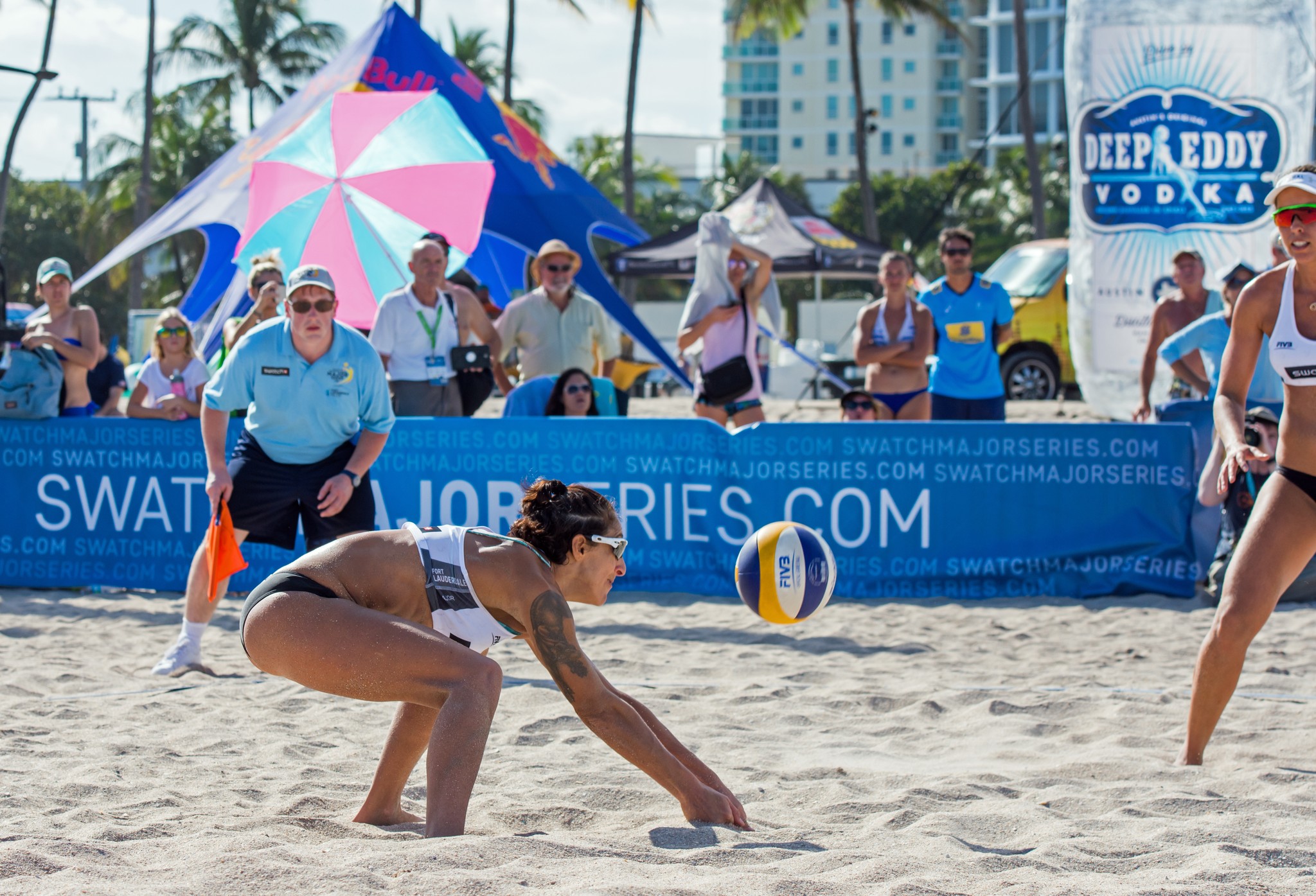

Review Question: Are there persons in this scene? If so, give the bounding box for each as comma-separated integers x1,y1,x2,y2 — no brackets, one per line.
16,256,128,416
366,232,622,416
149,267,396,672
852,252,934,421
1133,165,1316,769
216,251,286,417
238,478,750,839
915,230,1016,421
841,391,880,422
675,210,785,426
125,305,211,420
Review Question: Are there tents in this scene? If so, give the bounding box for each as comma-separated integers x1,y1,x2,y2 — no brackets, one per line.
597,174,885,300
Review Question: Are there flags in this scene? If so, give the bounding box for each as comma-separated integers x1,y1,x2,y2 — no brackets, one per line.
205,496,249,604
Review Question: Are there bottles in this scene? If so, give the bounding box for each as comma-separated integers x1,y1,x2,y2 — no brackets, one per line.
169,369,187,419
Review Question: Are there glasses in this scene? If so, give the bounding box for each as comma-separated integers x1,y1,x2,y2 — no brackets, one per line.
845,399,873,410
547,264,571,272
567,384,590,394
583,534,628,561
727,259,747,271
256,281,284,293
947,248,971,257
1272,204,1316,227
1227,277,1251,290
287,298,333,314
157,326,189,339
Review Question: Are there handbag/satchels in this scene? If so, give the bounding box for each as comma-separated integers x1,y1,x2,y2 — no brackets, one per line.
701,355,754,405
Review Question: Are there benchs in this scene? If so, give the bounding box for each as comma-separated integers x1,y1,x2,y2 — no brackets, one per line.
811,378,865,399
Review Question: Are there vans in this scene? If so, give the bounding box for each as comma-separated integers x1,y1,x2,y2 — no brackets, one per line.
983,238,1076,402
5,302,37,330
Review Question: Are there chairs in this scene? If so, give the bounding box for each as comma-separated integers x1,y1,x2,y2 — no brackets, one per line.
503,373,619,416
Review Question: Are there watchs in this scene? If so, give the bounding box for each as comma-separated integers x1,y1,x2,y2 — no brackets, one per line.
342,469,361,487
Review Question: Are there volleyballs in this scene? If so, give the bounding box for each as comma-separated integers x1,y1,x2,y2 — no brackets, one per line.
735,520,837,625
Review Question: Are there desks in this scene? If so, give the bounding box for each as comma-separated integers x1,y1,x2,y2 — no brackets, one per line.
819,355,937,401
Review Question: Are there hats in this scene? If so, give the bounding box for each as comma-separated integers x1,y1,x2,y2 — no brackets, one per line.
286,264,336,298
1264,172,1316,206
37,257,73,285
530,239,582,283
1244,405,1279,424
840,386,873,406
1172,251,1201,263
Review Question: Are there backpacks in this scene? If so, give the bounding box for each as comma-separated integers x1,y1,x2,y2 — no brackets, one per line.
0,343,65,420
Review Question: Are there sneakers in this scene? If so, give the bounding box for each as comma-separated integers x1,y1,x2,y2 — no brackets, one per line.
151,638,201,676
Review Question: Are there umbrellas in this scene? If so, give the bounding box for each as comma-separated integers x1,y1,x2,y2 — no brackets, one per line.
228,88,495,330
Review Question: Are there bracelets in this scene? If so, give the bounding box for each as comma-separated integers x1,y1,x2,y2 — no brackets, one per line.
251,305,263,318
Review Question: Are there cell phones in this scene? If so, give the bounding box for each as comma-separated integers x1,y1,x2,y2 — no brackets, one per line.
729,301,739,307
